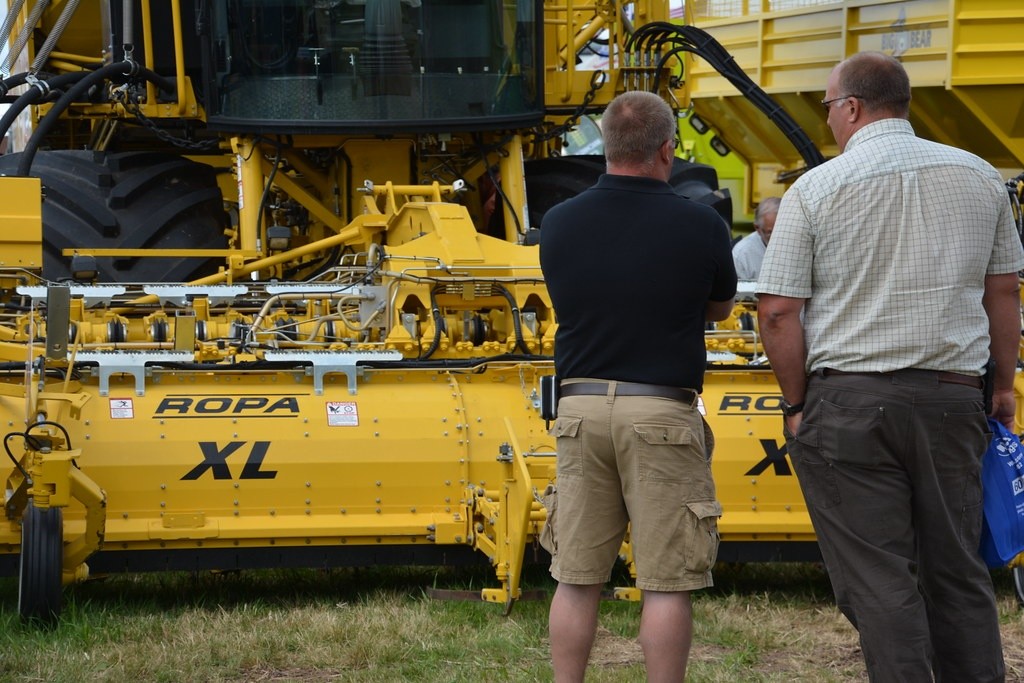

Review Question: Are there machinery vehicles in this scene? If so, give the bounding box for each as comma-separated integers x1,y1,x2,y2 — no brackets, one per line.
0,0,1024,623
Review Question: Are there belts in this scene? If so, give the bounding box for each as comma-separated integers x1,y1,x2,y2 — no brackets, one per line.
557,380,699,405
816,366,987,393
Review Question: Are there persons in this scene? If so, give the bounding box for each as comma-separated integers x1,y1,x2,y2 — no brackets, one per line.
731,197,782,282
540,90,739,681
756,52,1024,683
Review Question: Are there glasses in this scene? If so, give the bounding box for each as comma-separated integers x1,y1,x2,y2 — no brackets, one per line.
821,94,863,112
660,138,680,150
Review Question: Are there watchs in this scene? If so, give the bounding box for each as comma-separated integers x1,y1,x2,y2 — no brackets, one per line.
781,397,805,416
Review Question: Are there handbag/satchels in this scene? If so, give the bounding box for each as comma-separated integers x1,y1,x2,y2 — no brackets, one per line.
985,416,1024,572
